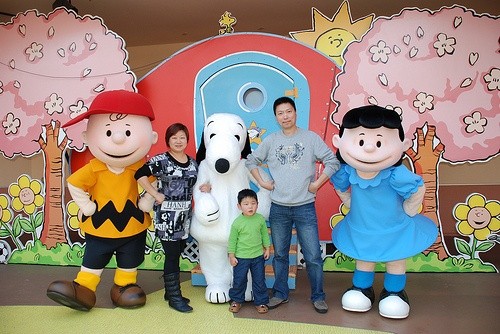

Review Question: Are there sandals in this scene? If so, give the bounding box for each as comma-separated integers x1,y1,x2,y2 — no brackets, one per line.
256,305,268,313
229,301,240,312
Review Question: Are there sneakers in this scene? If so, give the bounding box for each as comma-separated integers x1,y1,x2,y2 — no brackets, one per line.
313,301,328,313
266,297,288,308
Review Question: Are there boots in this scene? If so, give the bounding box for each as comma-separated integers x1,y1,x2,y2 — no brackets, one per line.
164,272,193,312
164,267,190,302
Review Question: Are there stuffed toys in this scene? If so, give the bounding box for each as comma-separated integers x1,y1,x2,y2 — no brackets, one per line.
189,112,273,304
43,89,162,310
331,105,439,320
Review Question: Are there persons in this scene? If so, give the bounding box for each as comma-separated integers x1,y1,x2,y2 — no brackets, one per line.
246,97,343,314
225,189,272,315
133,122,197,312
